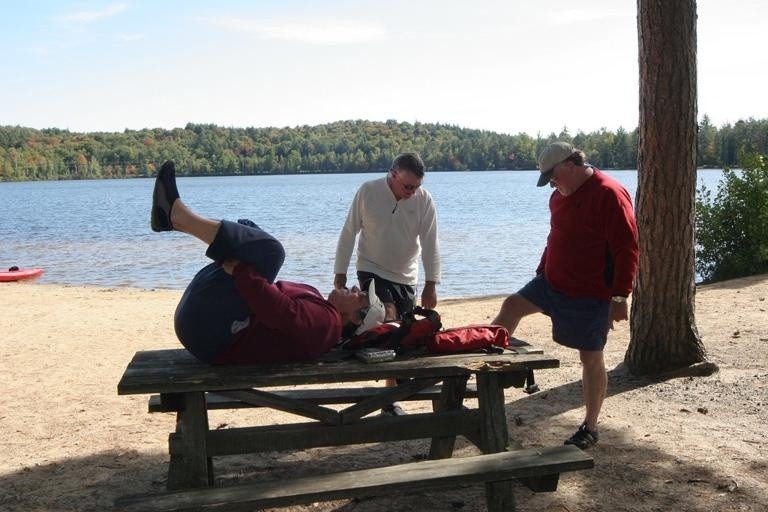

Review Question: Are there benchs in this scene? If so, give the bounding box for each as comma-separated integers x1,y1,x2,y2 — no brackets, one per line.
147,381,479,485
114,443,595,511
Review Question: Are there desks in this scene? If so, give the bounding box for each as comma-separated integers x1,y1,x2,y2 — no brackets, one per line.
118,335,561,510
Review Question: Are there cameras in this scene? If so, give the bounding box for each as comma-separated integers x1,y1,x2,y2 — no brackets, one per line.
355,347,397,364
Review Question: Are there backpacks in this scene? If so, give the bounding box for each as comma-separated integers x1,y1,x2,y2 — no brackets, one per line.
360,304,442,355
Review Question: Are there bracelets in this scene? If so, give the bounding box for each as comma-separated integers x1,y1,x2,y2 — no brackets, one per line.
611,295,626,303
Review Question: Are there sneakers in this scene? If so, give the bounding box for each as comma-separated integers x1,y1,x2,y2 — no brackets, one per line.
564,424,599,451
151,160,180,233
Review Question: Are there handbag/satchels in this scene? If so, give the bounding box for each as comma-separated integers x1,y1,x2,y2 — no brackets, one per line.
430,324,509,354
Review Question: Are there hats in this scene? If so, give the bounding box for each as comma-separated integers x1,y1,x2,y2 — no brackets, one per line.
536,141,576,187
351,278,386,337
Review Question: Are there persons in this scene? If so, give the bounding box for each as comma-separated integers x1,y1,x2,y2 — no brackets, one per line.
485,141,639,450
150,158,389,366
333,152,442,419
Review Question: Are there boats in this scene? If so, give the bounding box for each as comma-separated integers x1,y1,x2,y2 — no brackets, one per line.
0,267,44,281
